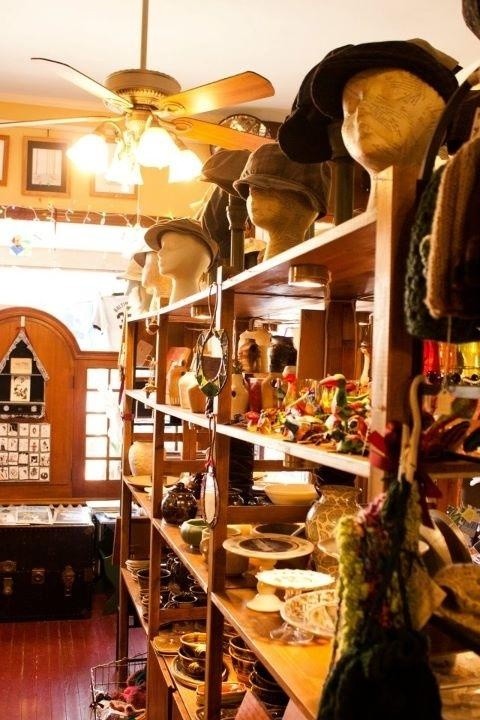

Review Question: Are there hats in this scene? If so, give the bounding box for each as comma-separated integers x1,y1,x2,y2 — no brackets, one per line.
233,143,331,221
144,217,219,274
276,38,458,164
198,148,252,200
116,245,159,283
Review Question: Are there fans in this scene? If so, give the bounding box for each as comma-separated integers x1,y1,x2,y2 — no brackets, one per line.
0,1,286,152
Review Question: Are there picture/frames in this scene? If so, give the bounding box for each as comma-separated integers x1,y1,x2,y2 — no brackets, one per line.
20,136,139,201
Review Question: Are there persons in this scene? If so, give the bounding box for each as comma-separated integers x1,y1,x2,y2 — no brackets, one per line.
241,180,319,268
156,230,213,307
335,68,447,212
118,270,152,318
140,251,172,306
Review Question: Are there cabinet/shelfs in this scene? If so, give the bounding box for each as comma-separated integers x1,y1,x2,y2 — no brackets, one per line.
0,524,94,622
113,143,479,720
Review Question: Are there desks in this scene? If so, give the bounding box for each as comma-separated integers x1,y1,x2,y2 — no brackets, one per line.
94,511,121,577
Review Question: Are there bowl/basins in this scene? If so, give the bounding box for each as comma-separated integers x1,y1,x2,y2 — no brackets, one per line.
124,552,308,720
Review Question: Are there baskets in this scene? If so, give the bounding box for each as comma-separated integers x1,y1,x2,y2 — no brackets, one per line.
90,650,148,720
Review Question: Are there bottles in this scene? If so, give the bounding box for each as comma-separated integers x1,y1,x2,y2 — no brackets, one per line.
128,440,211,554
